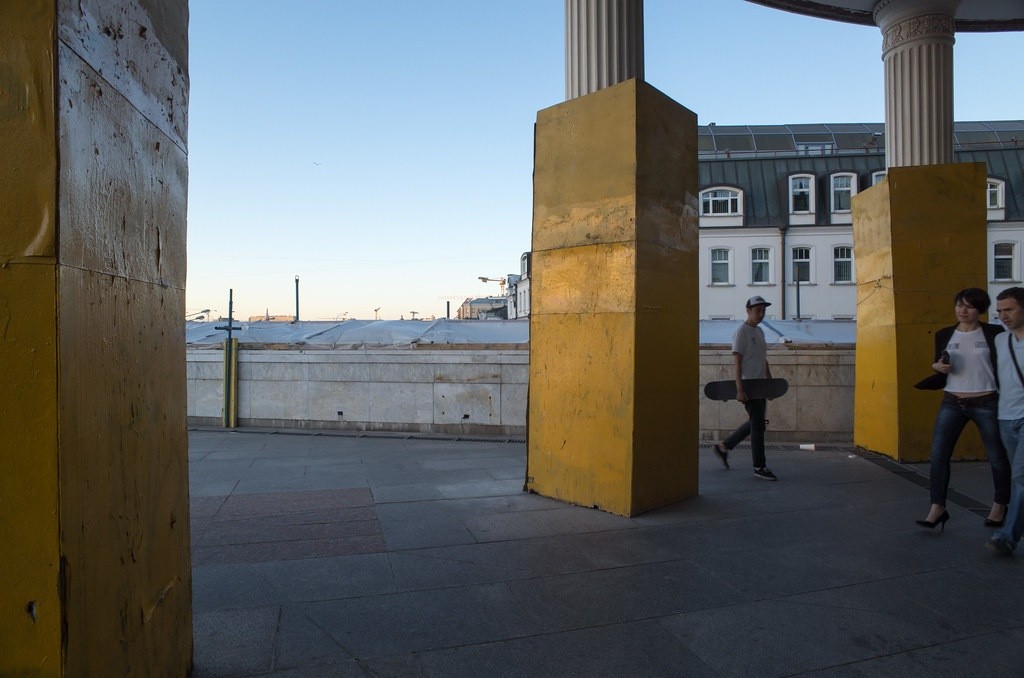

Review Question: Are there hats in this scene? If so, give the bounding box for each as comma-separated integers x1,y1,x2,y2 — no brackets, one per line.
746,296,772,308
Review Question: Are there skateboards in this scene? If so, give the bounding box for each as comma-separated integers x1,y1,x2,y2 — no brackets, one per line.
703,377,790,402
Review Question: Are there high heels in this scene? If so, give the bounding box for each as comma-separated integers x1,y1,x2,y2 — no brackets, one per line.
916,509,950,533
983,506,1008,529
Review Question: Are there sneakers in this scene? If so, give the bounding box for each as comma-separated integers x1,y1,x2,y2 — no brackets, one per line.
753,466,777,480
712,444,730,470
985,533,1018,558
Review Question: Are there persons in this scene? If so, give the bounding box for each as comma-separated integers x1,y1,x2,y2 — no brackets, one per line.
913,288,1005,533
712,296,779,481
985,287,1024,555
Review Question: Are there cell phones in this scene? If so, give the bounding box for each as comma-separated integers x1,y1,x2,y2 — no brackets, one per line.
943,351,950,364
